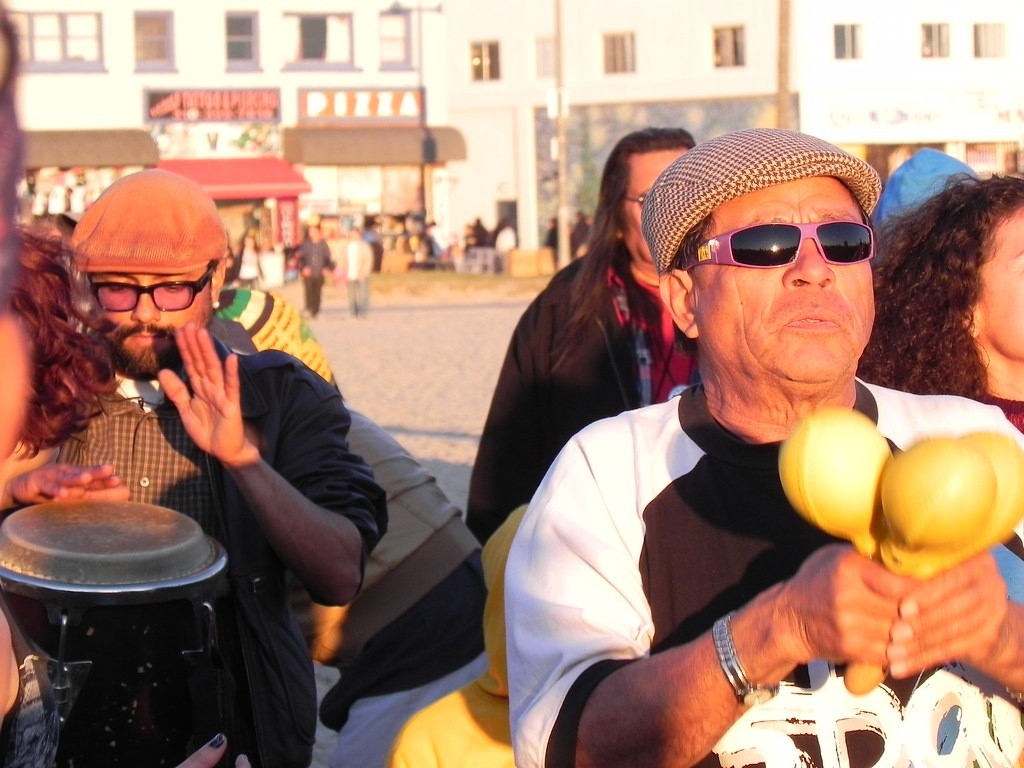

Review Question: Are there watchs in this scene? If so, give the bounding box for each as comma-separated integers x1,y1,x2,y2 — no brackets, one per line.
712,610,780,705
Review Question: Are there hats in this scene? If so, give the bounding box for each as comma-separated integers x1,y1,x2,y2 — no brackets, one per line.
641,128,882,278
69,168,227,274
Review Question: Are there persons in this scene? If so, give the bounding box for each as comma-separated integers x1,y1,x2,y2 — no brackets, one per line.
296,222,337,317
465,217,518,274
856,176,1024,436
503,129,1024,768
5,169,389,768
386,502,528,768
543,212,594,259
0,229,120,768
869,147,978,267
285,408,488,768
405,222,439,270
223,233,263,291
465,127,701,549
343,227,373,314
364,221,385,273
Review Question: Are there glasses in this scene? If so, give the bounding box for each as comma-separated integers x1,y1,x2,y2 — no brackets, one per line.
623,192,647,207
677,221,874,271
88,264,216,312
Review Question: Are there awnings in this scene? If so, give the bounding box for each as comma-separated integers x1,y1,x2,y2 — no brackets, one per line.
158,159,312,200
21,129,160,168
285,128,467,164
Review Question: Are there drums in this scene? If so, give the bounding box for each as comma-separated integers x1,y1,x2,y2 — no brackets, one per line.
1,498,232,768
